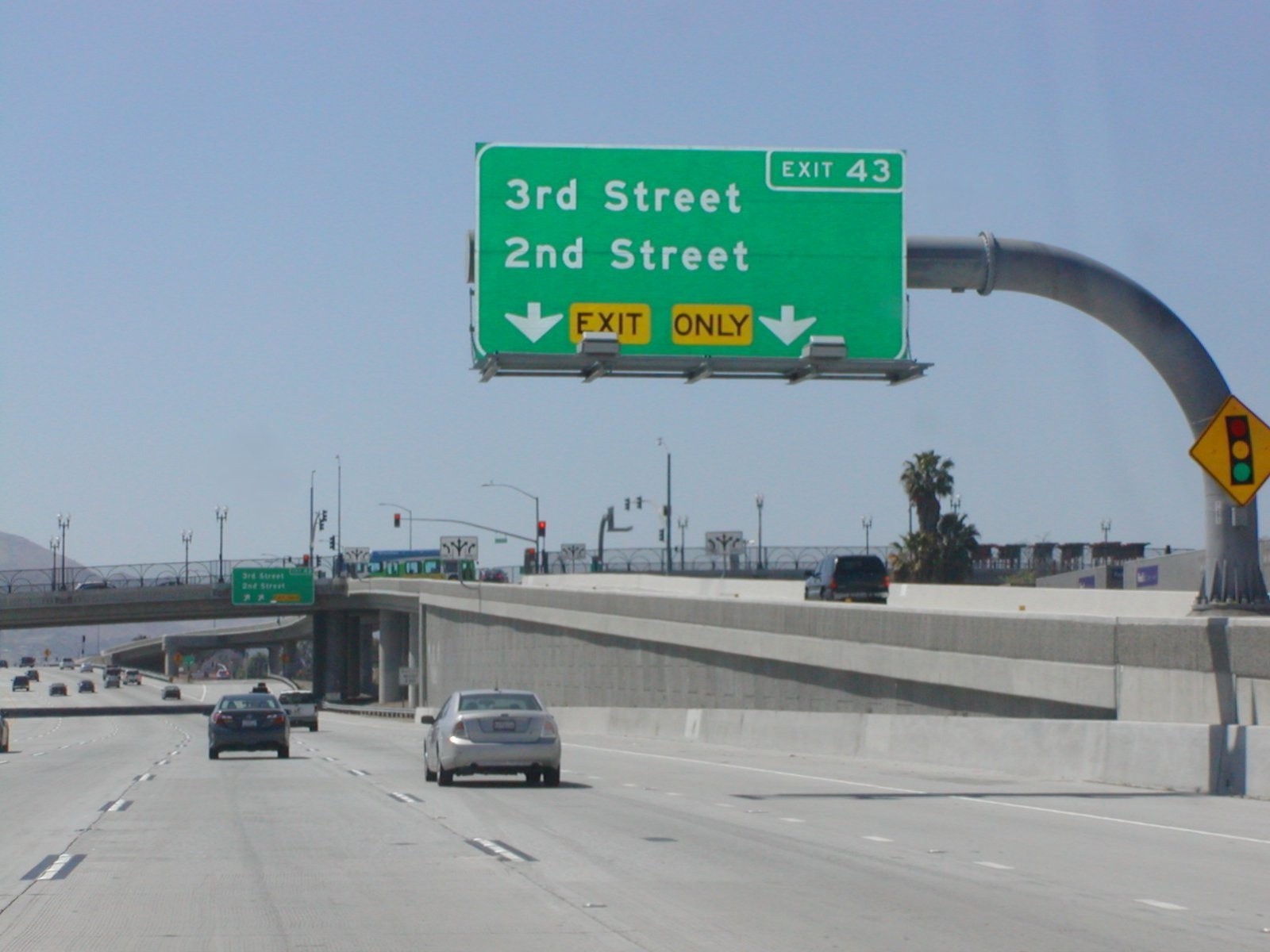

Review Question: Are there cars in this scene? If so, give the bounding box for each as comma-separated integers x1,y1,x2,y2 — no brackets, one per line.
419,688,562,788
161,686,181,700
0,653,142,688
48,683,67,696
0,710,10,754
201,693,292,760
77,680,95,693
214,663,230,680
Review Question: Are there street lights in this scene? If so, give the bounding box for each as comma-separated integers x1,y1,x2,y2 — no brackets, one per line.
755,492,765,569
483,483,539,575
182,505,229,586
380,502,412,551
47,514,70,591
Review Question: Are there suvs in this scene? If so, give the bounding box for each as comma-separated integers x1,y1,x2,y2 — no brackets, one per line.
74,582,117,591
277,690,319,732
804,554,890,604
10,675,30,692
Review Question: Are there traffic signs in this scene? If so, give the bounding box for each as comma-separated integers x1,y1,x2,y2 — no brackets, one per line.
232,567,315,606
469,143,908,372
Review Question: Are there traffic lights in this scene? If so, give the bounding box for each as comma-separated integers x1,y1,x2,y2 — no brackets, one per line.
394,513,400,528
625,498,630,510
658,528,664,541
637,496,641,509
303,509,335,570
538,522,545,536
529,548,534,561
